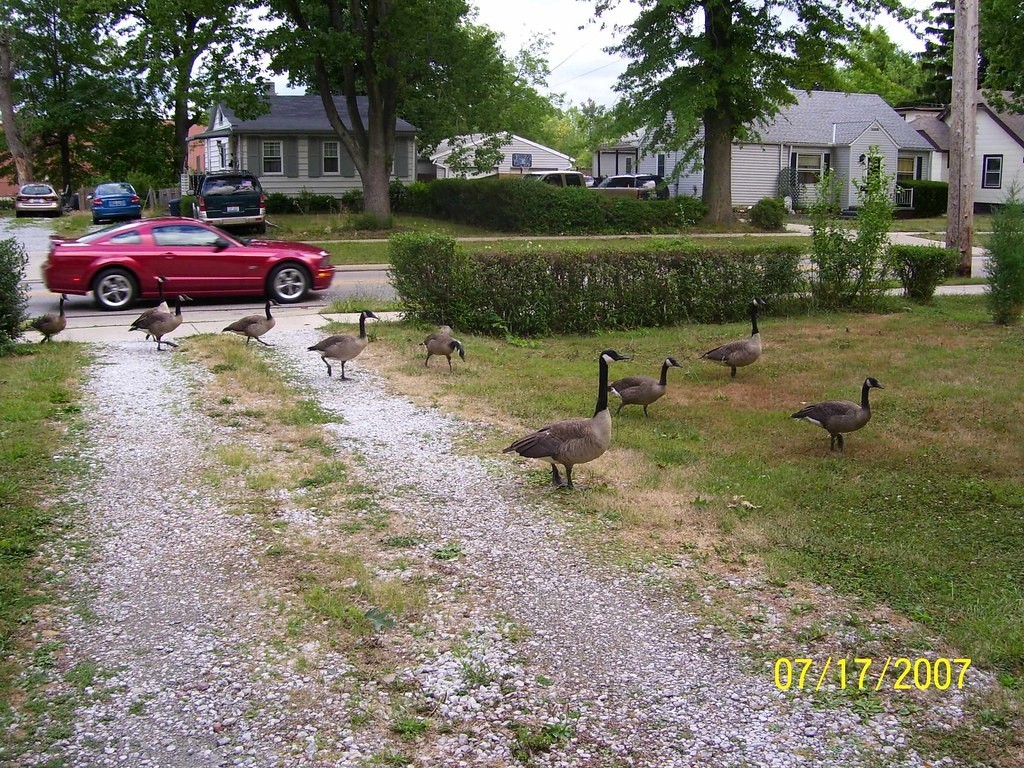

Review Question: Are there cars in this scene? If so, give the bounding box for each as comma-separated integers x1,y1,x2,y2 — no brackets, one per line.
41,216,335,311
11,183,63,218
87,182,142,224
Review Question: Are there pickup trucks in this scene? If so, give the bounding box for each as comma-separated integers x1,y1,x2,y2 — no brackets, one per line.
185,169,269,235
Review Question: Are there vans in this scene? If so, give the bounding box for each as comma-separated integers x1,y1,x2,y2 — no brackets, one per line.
520,170,670,202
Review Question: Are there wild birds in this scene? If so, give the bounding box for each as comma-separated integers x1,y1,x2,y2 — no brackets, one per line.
23,293,71,344
127,274,884,493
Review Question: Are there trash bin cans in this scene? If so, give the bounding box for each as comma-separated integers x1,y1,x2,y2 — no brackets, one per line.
167,199,182,216
